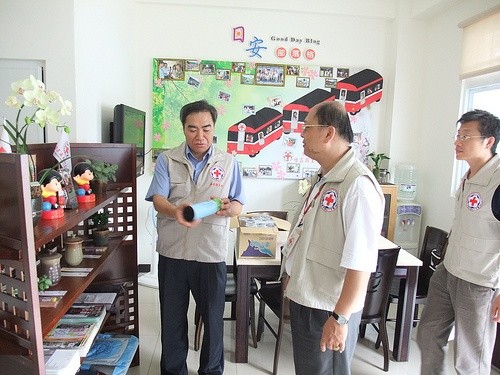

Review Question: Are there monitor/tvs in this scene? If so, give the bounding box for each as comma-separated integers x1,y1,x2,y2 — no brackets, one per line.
110,104,146,178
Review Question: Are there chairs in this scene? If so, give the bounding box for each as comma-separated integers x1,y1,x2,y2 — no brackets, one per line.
194,273,258,351
361,246,401,372
360,225,449,349
256,246,290,375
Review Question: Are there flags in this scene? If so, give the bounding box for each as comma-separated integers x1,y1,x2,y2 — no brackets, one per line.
0,120,12,153
53,126,73,174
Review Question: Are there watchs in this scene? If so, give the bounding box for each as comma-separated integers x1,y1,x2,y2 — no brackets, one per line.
331,310,350,325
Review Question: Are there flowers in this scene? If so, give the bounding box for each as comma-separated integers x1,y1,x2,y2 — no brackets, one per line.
298,178,311,195
0,75,89,184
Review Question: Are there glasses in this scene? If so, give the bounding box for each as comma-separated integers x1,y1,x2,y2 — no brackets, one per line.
301,124,335,132
454,135,489,141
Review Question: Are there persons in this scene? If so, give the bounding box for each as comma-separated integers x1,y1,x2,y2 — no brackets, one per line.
145,100,246,375
278,101,386,375
73,162,95,195
38,170,67,210
415,109,500,375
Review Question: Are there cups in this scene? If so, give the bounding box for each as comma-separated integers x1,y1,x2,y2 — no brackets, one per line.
64,234,85,266
37,249,63,287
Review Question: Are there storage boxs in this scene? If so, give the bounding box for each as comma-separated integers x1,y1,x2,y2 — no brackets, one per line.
230,213,292,260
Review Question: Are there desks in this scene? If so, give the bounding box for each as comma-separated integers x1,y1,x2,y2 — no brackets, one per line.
235,229,423,363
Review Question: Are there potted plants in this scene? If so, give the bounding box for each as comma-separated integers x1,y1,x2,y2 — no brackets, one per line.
91,209,111,246
368,152,391,184
85,159,120,194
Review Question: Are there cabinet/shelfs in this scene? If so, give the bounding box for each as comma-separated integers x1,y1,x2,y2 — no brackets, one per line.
0,143,140,375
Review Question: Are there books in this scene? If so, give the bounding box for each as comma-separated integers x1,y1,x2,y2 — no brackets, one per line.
42,293,117,375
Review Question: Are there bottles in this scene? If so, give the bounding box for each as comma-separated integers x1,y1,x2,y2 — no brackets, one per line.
395,164,417,203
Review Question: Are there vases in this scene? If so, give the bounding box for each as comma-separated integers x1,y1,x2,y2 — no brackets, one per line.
30,182,42,227
